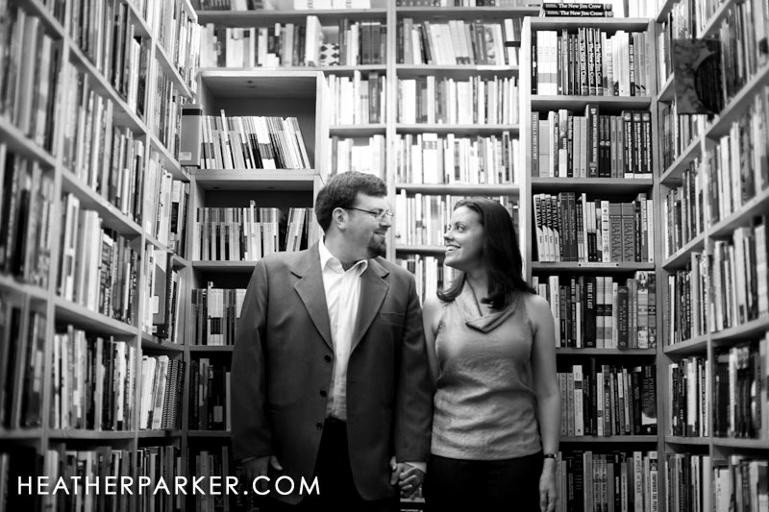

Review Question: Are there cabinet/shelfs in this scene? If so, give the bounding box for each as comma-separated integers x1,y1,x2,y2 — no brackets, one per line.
1,0,769,512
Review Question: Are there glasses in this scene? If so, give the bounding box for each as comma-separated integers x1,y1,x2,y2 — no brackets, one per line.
352,206,395,223
